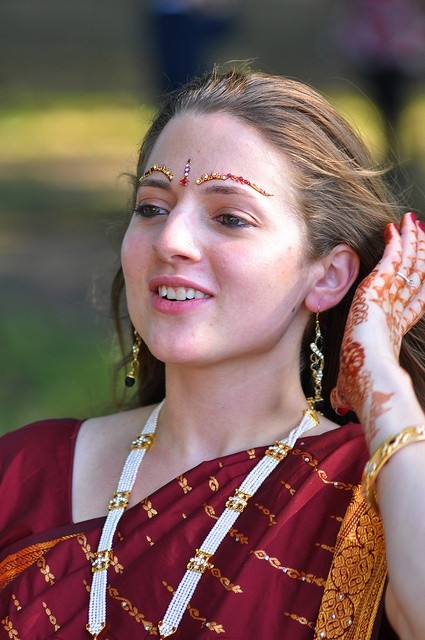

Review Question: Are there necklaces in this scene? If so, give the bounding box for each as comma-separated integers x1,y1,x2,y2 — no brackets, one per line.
84,397,320,640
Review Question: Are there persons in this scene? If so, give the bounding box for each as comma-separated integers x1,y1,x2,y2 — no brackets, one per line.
0,62,425,640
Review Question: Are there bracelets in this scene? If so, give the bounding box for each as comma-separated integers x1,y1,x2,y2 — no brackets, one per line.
361,424,425,508
